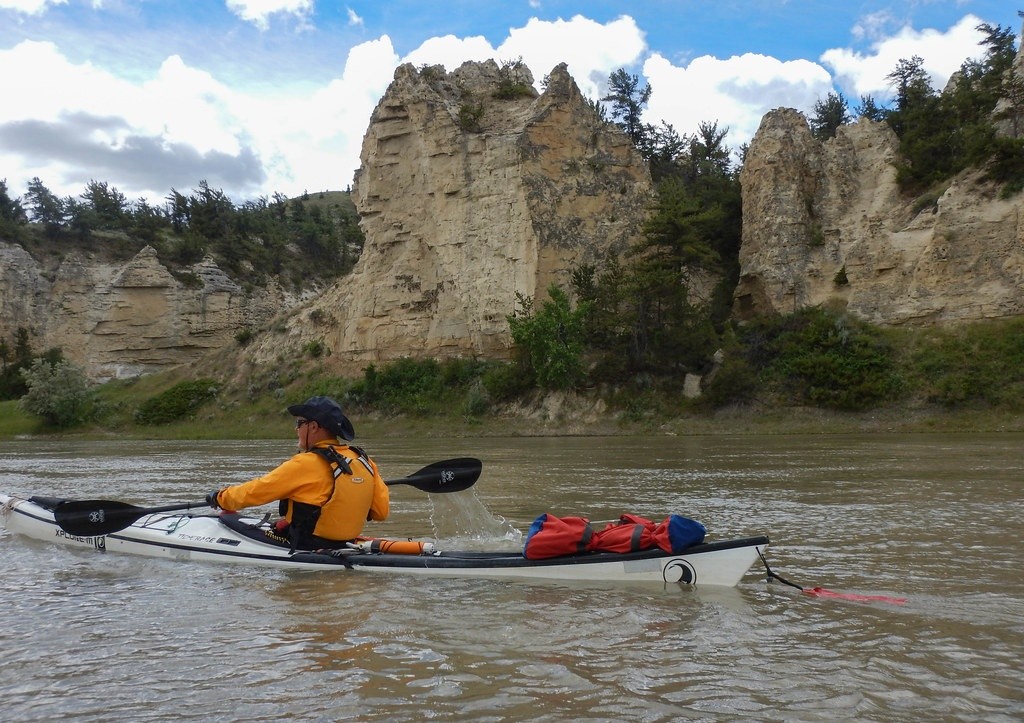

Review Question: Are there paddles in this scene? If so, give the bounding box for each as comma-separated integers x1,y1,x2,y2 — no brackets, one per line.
51,457,483,538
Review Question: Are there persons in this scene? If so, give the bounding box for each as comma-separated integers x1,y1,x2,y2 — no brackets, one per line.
207,396,390,550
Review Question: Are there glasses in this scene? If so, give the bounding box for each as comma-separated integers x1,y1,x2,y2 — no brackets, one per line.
295,419,312,428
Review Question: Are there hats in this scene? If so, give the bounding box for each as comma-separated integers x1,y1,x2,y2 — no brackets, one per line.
288,397,355,442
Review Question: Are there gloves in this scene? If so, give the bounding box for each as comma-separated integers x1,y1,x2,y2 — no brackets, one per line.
205,492,219,511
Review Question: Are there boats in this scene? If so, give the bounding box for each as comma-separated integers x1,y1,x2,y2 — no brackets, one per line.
0,493,769,588
